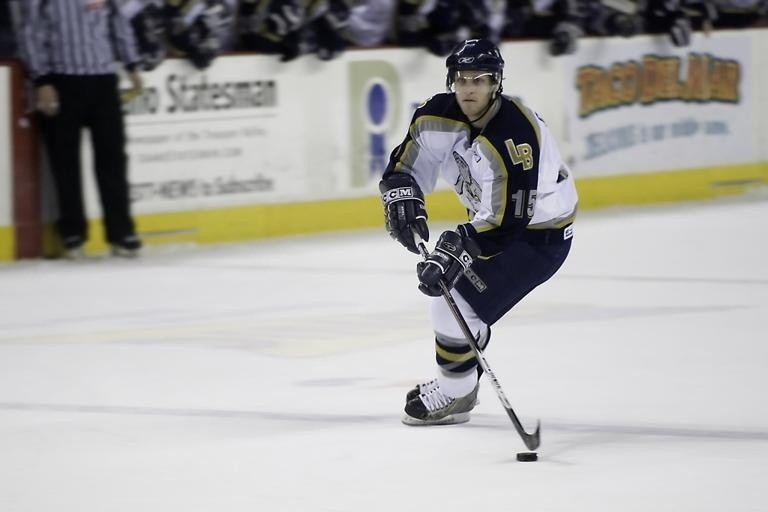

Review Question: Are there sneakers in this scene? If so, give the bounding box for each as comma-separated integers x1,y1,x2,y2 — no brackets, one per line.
56,224,140,250
406,379,480,419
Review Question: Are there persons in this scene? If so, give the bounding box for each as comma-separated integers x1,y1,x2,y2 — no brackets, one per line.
379,38,579,422
119,0,768,70
5,0,145,251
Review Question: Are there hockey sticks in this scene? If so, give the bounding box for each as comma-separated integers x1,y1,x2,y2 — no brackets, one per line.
407,223,540,450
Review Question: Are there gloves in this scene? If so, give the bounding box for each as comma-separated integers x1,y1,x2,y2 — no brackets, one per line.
418,230,475,298
380,174,429,254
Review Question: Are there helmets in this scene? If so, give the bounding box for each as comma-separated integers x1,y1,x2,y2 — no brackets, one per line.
446,38,504,97
